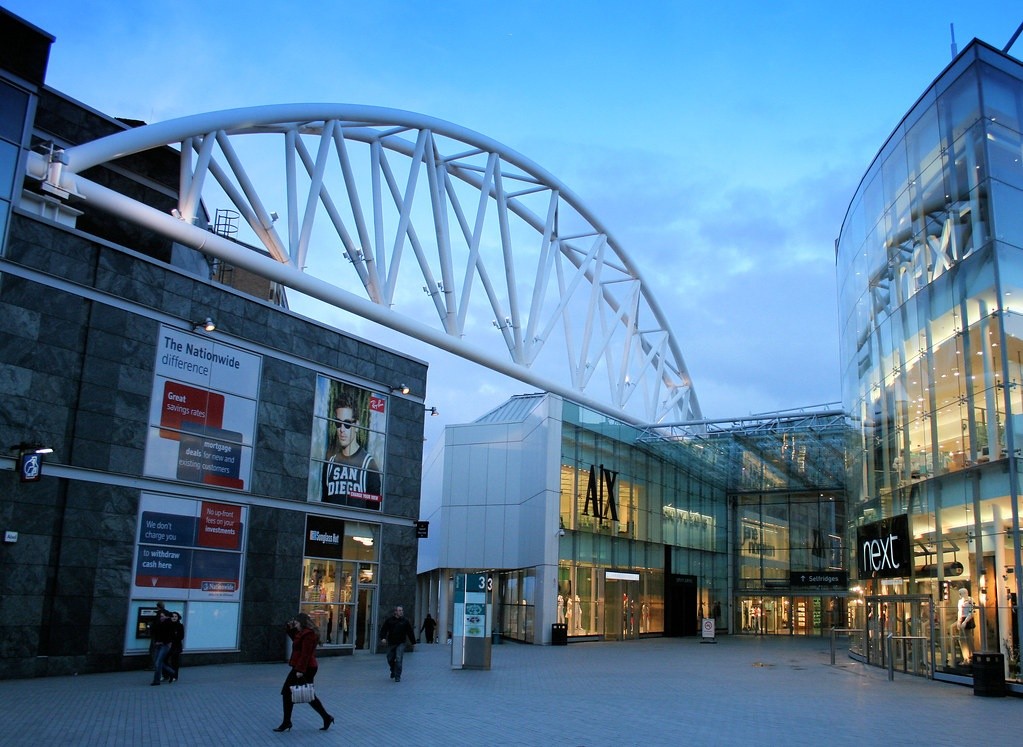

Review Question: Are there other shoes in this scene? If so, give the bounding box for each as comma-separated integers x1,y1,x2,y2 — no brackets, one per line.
395,676,400,682
160,677,169,682
168,671,176,683
391,672,395,678
151,679,160,686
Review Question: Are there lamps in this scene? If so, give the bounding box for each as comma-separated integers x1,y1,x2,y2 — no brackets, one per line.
9,443,54,456
192,317,216,332
390,382,410,395
426,407,439,416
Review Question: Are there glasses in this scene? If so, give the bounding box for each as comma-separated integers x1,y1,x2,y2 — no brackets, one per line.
335,418,357,429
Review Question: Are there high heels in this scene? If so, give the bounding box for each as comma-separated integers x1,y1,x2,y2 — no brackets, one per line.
319,716,334,730
273,723,292,732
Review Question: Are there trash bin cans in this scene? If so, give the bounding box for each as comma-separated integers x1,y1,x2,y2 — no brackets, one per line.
973,649,1007,699
551,623,568,647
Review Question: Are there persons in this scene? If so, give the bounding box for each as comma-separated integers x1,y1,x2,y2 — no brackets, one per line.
624,594,650,632
420,614,438,644
379,605,416,682
744,603,749,629
148,601,185,687
557,594,565,628
273,615,335,732
565,594,583,630
326,573,347,645
753,605,762,630
956,588,976,665
322,393,381,511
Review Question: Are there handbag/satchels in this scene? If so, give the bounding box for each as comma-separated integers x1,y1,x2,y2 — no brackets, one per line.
290,676,315,703
